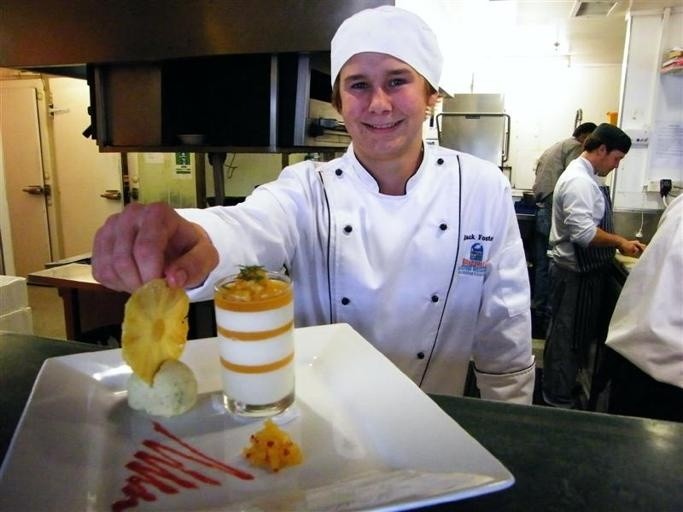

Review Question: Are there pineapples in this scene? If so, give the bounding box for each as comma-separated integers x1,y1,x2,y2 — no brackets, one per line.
120,279,189,387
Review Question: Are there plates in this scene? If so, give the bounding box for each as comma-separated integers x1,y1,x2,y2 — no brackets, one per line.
0,322,519,512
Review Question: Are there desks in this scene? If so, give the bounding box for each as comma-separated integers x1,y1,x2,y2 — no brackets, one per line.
26,250,127,347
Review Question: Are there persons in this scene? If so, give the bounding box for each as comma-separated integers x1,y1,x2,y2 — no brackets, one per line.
531,122,596,328
91,5,536,407
603,190,682,423
541,122,646,409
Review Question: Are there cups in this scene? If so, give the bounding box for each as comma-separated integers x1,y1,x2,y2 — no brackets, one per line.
211,269,296,418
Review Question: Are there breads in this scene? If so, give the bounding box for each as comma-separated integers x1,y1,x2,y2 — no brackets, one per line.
127,360,197,416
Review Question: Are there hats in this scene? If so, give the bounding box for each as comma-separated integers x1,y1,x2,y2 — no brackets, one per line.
330,5,445,93
592,123,632,155
